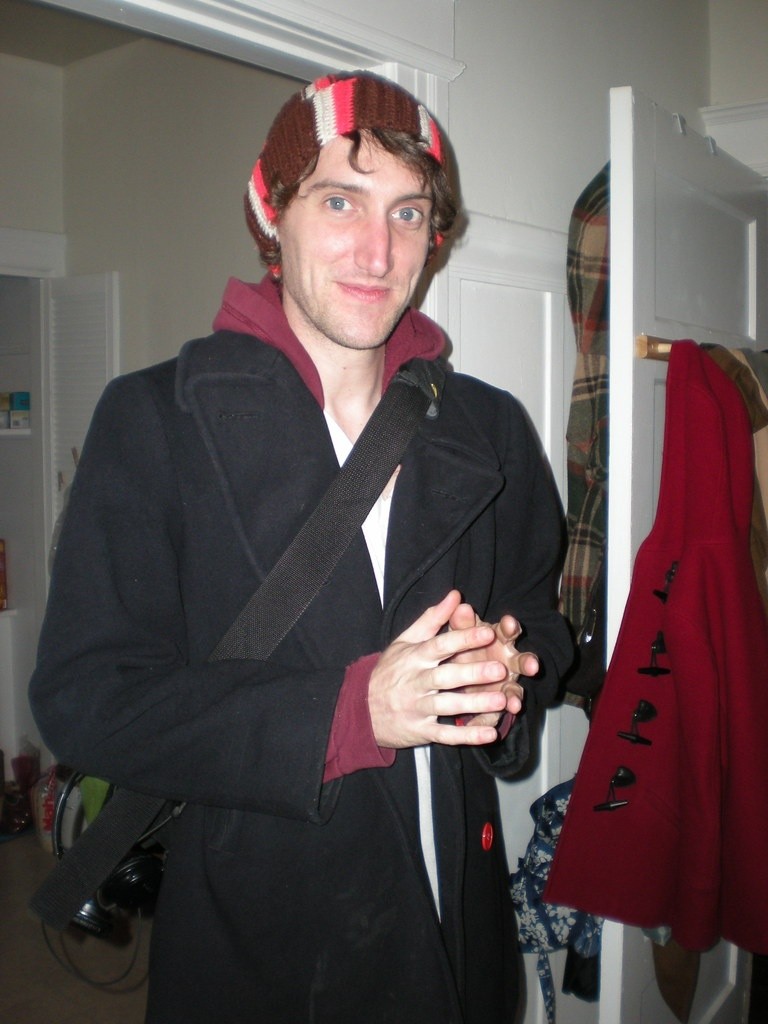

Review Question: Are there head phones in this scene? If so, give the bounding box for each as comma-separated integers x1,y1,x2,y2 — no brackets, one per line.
52,767,165,951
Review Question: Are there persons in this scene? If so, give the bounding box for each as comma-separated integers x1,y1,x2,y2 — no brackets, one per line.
28,72,578,1024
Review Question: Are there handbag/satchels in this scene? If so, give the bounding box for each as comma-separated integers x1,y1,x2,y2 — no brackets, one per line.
508,777,603,1004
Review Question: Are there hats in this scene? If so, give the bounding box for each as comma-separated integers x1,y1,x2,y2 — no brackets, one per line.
244,69,451,284
